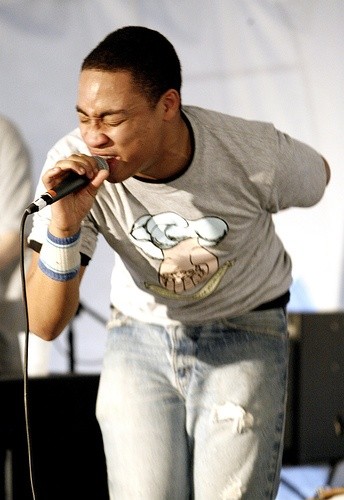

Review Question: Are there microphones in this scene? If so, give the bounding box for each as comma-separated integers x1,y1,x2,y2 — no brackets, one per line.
25,156,109,214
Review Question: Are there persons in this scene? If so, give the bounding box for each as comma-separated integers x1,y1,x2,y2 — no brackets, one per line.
22,24,330,500
0,112,37,500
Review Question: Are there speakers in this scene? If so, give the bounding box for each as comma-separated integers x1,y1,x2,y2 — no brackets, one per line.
282,311,344,465
0,374,110,500
